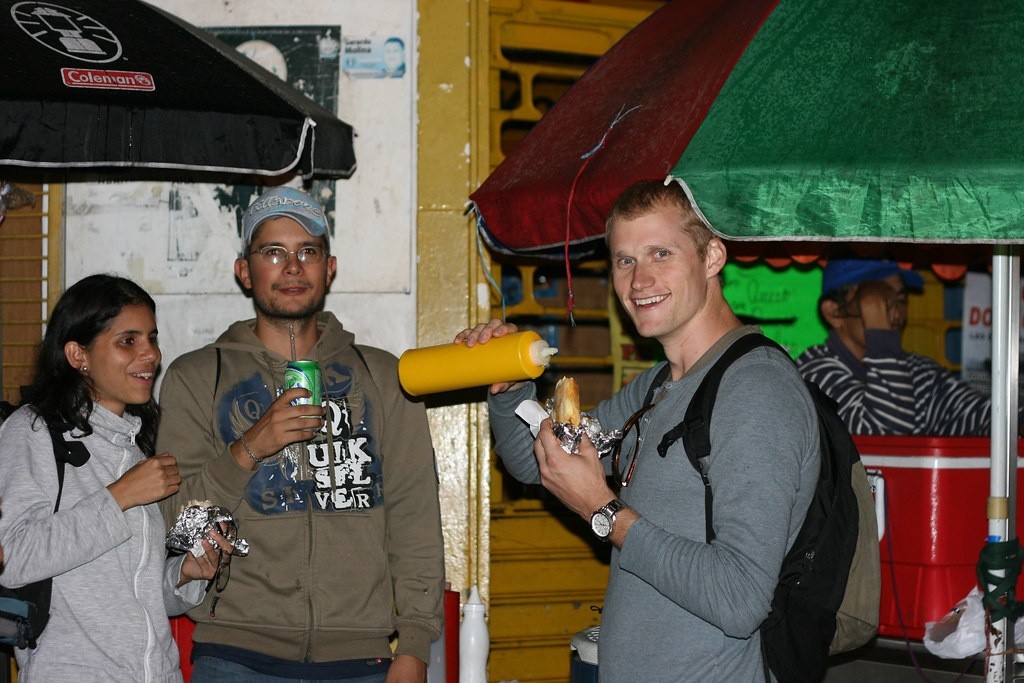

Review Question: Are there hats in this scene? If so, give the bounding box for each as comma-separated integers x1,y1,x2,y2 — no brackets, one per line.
821,256,924,299
243,186,330,248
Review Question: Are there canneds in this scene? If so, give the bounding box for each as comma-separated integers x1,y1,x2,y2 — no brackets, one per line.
283,359,322,432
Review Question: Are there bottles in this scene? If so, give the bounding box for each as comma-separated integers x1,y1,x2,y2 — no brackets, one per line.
398,329,559,396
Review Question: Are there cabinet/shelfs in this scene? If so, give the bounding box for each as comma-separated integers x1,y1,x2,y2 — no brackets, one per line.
490,29,623,511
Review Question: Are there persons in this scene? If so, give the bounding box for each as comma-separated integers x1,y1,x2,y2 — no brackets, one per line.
790,259,1024,437
0,272,233,683
453,180,821,683
156,188,446,683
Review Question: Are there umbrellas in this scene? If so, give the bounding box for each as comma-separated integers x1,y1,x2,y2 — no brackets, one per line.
0,0,357,189
462,0,1024,683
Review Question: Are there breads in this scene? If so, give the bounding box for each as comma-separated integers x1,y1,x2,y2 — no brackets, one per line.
555,376,581,430
179,498,214,513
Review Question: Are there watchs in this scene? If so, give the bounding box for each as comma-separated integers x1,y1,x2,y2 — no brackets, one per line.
589,497,628,543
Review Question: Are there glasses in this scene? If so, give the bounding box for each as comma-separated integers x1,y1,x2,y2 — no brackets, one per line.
205,520,238,593
247,246,331,263
611,404,654,487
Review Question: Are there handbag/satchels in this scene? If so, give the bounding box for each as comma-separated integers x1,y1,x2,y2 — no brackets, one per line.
0,577,53,650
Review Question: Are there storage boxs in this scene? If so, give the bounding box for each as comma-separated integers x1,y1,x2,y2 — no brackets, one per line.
850,434,1024,643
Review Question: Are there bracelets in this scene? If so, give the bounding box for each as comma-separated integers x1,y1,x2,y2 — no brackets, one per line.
241,431,262,462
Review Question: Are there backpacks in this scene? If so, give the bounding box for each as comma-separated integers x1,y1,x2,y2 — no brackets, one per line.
644,332,880,682
